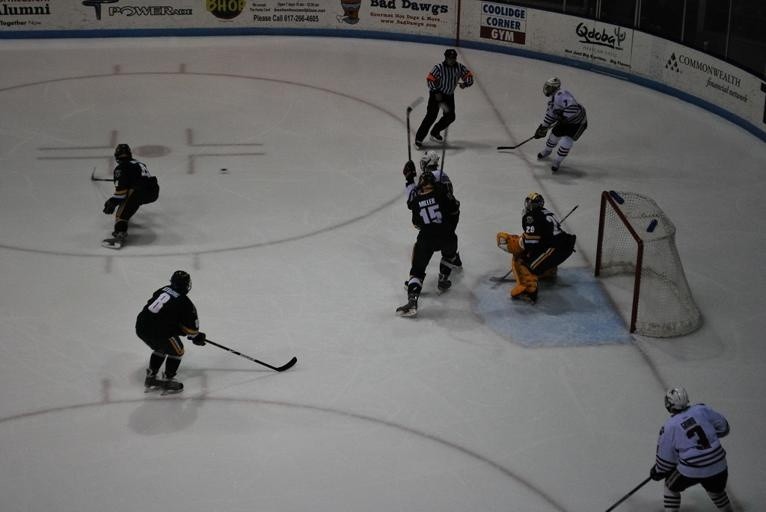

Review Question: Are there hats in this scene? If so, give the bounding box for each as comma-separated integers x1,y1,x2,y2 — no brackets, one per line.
444,49,457,58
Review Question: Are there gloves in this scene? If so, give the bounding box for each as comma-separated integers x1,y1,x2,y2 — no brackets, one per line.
178,328,206,346
103,199,118,214
403,160,416,180
535,124,548,139
650,463,669,481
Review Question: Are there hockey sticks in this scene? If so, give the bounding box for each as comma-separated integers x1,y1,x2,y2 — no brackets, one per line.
497,122,556,149
406,96,424,161
91,166,114,181
489,205,579,282
187,335,297,372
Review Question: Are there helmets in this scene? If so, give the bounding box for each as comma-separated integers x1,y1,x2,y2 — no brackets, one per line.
170,270,192,295
543,77,561,97
522,192,544,215
114,144,132,164
664,385,689,413
418,150,440,193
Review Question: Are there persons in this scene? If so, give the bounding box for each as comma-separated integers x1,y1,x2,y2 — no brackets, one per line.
414,49,474,150
497,192,574,305
536,75,587,171
103,145,159,246
395,173,460,314
401,151,462,269
136,271,205,392
652,385,733,511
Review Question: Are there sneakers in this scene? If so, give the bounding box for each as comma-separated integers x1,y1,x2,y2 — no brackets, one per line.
452,251,462,266
438,273,451,290
396,298,417,313
415,140,423,147
430,130,442,140
551,160,561,172
145,368,184,390
537,149,552,159
103,231,128,247
